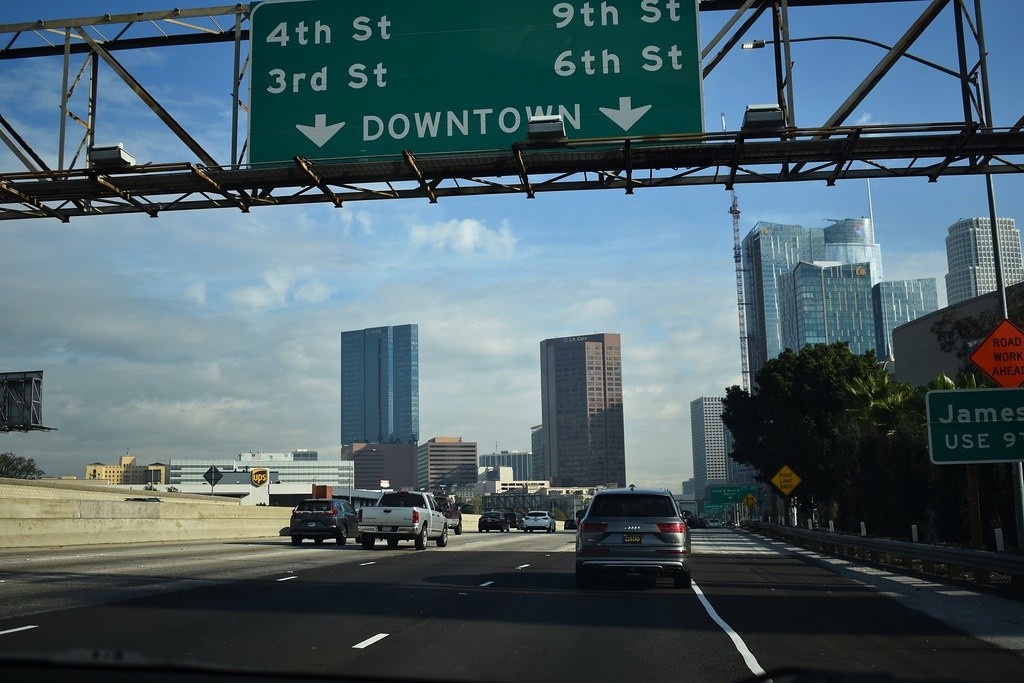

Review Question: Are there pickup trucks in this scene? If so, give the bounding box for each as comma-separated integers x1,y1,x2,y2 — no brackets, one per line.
357,491,448,550
434,497,462,535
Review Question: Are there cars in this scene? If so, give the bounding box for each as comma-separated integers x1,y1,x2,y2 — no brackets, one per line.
290,499,361,545
576,484,694,589
504,512,524,530
522,510,556,532
479,511,510,533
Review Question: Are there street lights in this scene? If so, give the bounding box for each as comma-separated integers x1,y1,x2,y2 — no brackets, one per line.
742,36,1024,554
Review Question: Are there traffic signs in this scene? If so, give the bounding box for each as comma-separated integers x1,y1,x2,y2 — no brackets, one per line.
247,0,706,169
